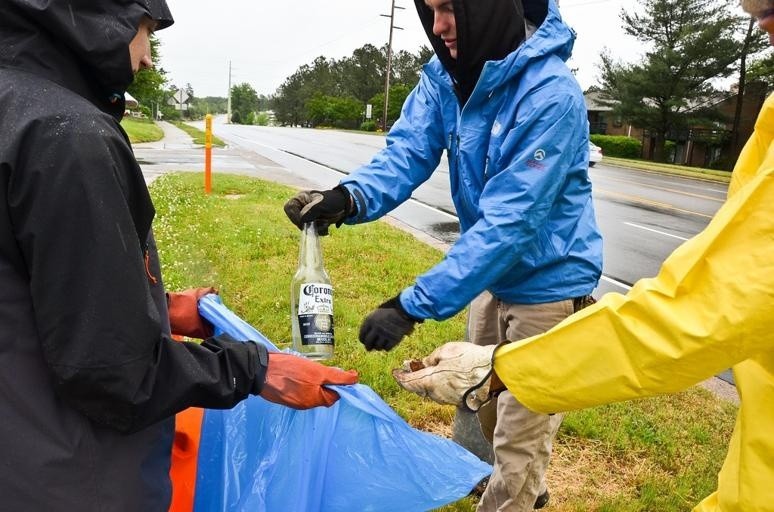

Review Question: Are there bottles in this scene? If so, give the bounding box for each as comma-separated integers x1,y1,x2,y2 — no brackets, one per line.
289,221,335,359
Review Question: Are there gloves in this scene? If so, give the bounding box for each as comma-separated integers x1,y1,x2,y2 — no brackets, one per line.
283,183,356,238
357,292,428,355
163,285,221,341
256,351,360,413
390,339,514,417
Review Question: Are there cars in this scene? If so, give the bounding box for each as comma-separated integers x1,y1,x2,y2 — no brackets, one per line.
587,138,604,167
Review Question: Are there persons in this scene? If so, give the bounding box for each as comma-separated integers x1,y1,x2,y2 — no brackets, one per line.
0,0,362,512
389,0,774,512
278,0,607,512
